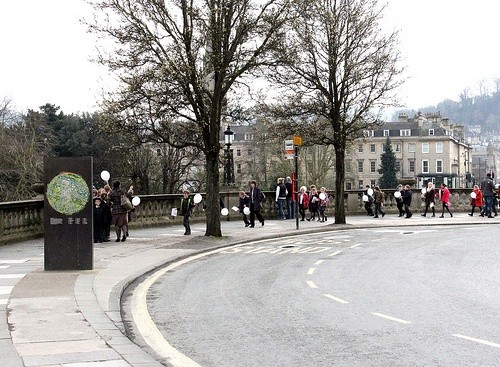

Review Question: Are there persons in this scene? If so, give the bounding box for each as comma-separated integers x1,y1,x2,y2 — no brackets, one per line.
480,173,500,218
435,183,453,218
364,185,386,218
276,176,296,220
421,181,435,217
249,180,265,228
394,192,401,198
237,191,250,227
297,184,328,223
468,185,482,216
395,184,413,218
92,181,134,243
177,190,195,235
202,197,225,214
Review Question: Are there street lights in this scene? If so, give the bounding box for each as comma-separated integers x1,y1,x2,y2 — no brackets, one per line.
223,124,235,186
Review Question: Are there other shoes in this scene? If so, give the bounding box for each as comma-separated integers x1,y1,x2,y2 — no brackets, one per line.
468,213,473,217
481,212,484,217
439,215,444,218
367,212,374,216
262,220,264,226
300,216,328,222
451,212,453,217
245,222,250,227
398,211,405,217
103,237,111,242
431,215,435,217
184,229,191,235
488,216,495,218
373,215,378,218
116,236,126,243
382,213,385,217
421,213,426,217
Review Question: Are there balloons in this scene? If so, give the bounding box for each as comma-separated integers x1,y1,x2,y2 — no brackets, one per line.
363,195,368,202
319,193,325,201
132,196,141,206
243,207,250,215
171,208,177,216
367,187,373,197
470,192,476,199
101,171,110,182
221,208,229,215
193,194,203,204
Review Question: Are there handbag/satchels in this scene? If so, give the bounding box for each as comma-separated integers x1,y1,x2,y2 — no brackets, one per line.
259,192,265,202
121,193,133,210
